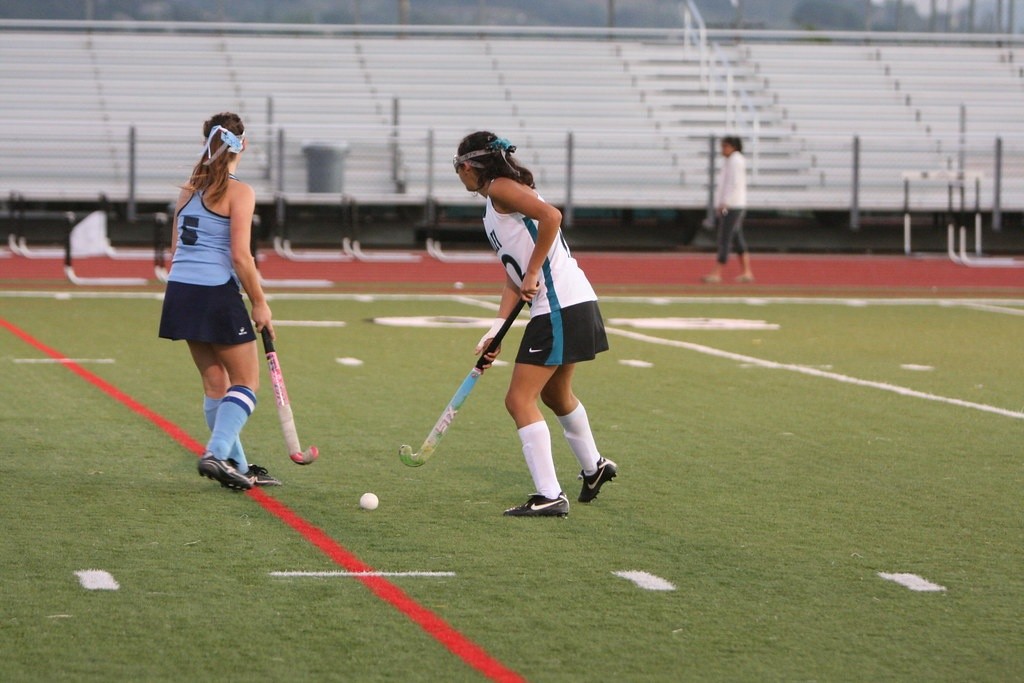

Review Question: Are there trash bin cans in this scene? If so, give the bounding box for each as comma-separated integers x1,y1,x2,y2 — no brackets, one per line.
302,138,350,192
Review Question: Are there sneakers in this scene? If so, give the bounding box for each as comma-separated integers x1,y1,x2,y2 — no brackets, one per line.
197,451,281,490
508,457,620,517
700,273,753,282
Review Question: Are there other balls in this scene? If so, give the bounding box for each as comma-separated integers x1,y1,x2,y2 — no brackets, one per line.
359,493,379,510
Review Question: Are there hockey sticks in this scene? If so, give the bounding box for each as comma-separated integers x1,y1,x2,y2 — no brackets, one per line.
259,322,319,467
397,284,542,467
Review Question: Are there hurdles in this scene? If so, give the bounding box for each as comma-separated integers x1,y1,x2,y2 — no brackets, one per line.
1,173,984,263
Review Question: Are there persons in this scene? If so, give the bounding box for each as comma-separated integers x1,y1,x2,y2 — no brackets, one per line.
453,130,617,519
700,135,754,285
159,111,283,492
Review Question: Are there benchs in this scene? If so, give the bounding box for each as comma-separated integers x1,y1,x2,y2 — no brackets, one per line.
0,19,1023,215
